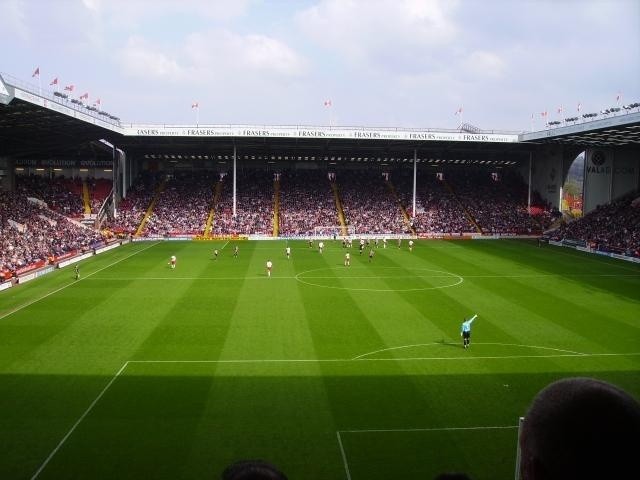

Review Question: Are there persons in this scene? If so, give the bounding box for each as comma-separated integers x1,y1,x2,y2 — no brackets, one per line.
0,170,639,285
461,313,477,349
519,377,640,480
222,461,286,480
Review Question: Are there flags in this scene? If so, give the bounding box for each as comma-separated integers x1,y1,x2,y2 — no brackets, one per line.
32,69,101,107
